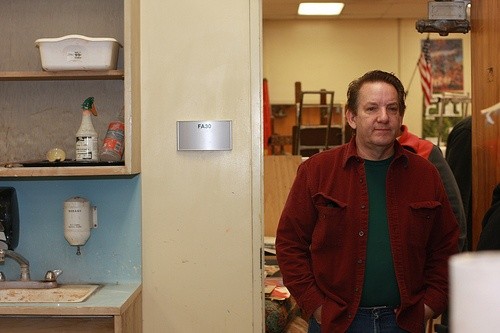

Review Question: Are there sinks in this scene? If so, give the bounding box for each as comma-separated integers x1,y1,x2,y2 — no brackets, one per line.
0,284,100,304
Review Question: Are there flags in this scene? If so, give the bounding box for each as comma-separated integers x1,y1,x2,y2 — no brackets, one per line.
416,38,434,108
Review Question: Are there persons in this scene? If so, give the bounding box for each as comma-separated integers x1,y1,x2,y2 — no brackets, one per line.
398,117,500,251
275,70,460,333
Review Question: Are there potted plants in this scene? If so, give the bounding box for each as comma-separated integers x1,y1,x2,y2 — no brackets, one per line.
423,117,455,158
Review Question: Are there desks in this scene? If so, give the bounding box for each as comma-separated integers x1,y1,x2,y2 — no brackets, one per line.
0,283,143,333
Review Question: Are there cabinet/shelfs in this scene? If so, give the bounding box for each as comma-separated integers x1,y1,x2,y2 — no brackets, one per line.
0,0,143,176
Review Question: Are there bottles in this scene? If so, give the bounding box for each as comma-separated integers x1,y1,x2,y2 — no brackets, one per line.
100,104,125,162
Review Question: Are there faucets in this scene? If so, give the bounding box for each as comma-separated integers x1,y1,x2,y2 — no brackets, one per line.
1,249,30,281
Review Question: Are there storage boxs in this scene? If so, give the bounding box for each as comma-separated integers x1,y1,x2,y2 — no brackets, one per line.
34,35,123,72
